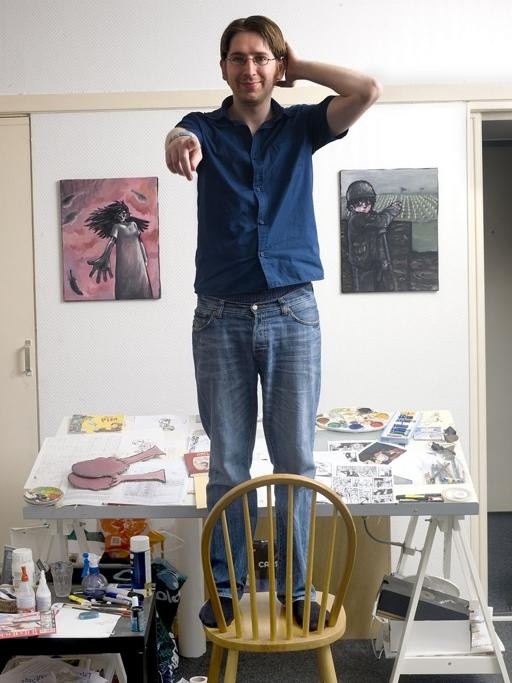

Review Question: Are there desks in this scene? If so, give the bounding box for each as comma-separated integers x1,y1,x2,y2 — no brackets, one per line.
21,408,509,683
0,584,157,683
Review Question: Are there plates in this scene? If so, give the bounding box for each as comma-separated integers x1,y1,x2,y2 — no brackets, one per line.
24,486,64,507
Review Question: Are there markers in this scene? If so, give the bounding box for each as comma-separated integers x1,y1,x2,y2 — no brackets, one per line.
105,592,143,606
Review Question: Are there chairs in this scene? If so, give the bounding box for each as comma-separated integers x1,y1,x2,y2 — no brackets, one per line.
201,473,355,683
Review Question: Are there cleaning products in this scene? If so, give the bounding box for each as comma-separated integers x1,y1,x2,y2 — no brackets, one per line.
80,552,108,602
15,566,35,614
35,569,52,614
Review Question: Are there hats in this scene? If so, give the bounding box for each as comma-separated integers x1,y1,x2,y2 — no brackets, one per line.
346,181,376,201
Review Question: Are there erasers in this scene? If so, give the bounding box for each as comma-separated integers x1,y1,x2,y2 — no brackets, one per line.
79,611,98,620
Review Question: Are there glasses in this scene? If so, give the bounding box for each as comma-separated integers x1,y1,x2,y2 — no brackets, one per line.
225,55,278,65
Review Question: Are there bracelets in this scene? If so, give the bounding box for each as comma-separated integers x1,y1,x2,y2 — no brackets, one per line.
166,130,200,146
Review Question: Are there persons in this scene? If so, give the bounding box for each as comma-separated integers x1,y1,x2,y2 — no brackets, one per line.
81,198,157,299
162,13,380,632
342,176,405,290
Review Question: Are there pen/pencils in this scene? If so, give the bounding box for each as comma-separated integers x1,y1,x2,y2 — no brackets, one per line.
102,597,130,605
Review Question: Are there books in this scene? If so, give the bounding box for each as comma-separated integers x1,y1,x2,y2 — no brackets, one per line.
184,450,210,478
375,609,402,621
378,572,471,624
0,608,58,639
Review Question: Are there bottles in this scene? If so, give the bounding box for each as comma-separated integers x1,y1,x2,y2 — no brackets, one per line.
36,570,52,611
131,606,145,631
130,535,153,598
51,560,74,597
14,565,37,611
11,548,35,590
